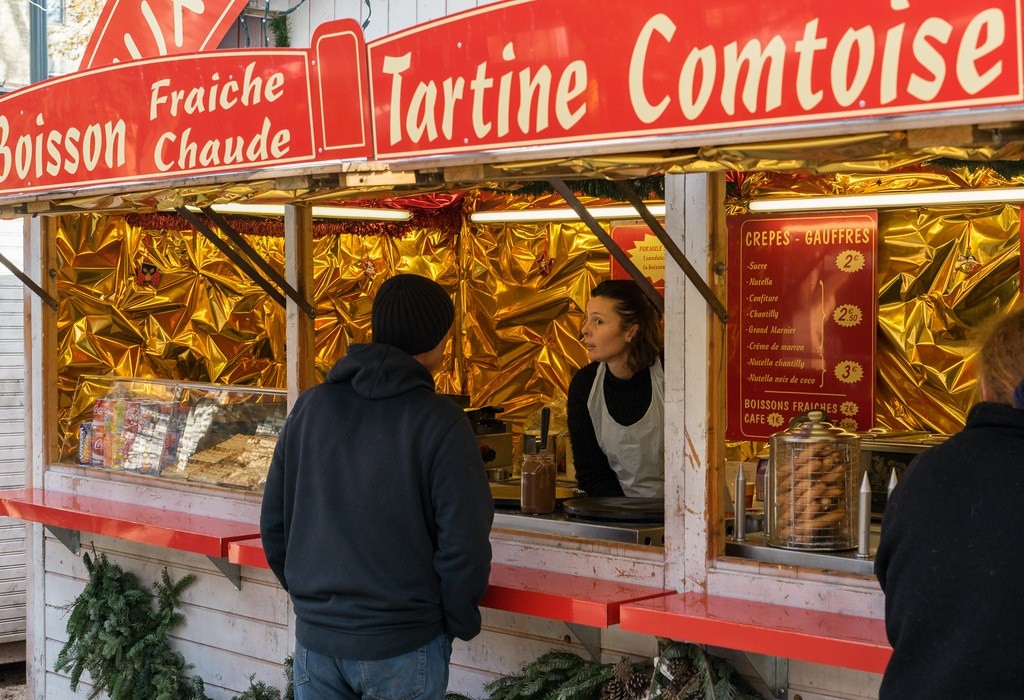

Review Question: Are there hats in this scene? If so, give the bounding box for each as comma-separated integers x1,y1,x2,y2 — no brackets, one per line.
372,275,457,354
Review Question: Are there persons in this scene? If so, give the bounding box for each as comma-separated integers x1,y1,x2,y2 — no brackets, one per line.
566,278,665,498
257,272,497,700
870,304,1024,700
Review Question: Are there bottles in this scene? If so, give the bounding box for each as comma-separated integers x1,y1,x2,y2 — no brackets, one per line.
521,455,557,513
